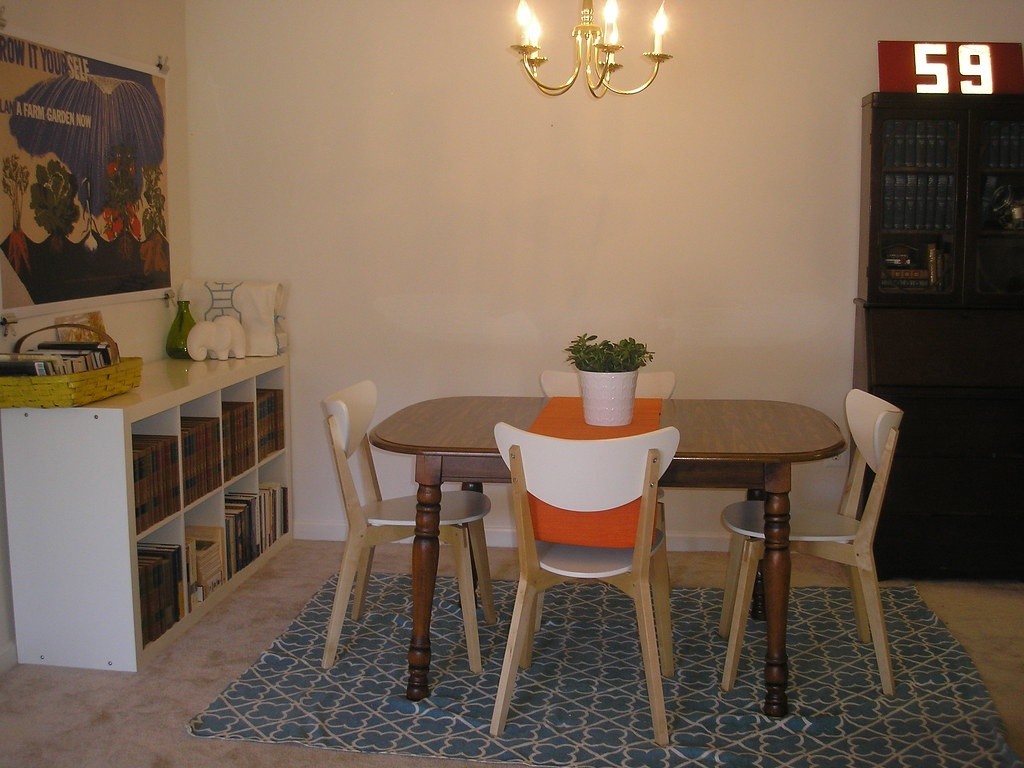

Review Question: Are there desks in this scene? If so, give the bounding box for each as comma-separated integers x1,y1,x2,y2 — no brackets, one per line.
369,397,850,719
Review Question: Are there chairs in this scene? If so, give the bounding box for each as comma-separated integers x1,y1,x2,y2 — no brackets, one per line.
720,389,907,697
320,380,500,674
489,419,678,746
538,370,673,596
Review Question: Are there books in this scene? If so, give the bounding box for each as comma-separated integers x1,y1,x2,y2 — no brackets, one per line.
137,541,183,650
878,234,985,296
255,386,290,462
221,397,258,484
180,415,223,508
224,482,291,581
881,118,1024,172
1,309,118,392
184,523,224,615
878,173,1003,231
131,433,180,536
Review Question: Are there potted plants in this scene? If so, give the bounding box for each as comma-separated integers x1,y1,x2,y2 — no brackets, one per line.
562,330,657,428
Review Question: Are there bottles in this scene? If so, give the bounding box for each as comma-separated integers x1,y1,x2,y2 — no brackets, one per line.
166,301,196,359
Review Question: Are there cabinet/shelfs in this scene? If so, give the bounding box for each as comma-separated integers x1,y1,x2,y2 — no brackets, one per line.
852,299,1023,585
858,93,1023,309
1,350,290,675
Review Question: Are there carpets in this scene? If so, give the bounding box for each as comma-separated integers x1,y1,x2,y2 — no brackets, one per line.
184,563,1024,768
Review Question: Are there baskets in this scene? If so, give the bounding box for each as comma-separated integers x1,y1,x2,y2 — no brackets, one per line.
0,324,143,409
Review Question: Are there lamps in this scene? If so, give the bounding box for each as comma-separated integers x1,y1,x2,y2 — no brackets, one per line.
505,0,677,99
